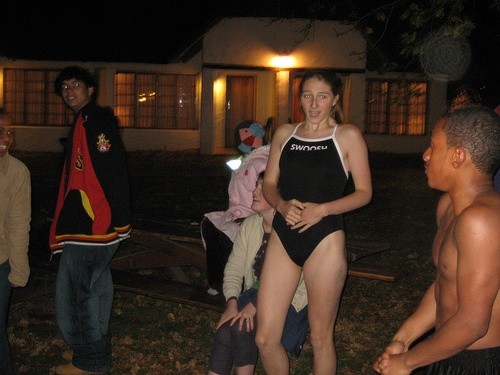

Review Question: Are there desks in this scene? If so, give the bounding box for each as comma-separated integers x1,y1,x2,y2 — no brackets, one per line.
129,208,390,273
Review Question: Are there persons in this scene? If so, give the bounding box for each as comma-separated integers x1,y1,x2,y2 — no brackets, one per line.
196,68,375,375
367,110,500,375
0,117,32,375
44,65,135,375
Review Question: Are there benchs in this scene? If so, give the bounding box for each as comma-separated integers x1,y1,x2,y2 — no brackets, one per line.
32,257,228,314
348,263,410,283
